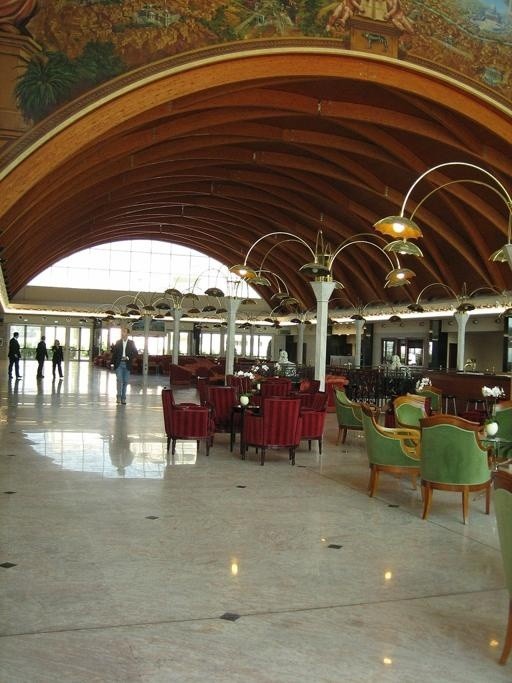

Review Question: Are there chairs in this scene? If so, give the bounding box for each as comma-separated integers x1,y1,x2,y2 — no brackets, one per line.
161,375,328,466
296,363,315,375
327,365,511,524
489,468,512,665
96,351,226,385
235,358,278,372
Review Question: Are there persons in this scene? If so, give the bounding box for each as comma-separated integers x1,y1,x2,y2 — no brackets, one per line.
109,327,137,405
36,336,48,378
52,340,64,377
326,0,364,33
8,332,22,378
384,0,416,36
